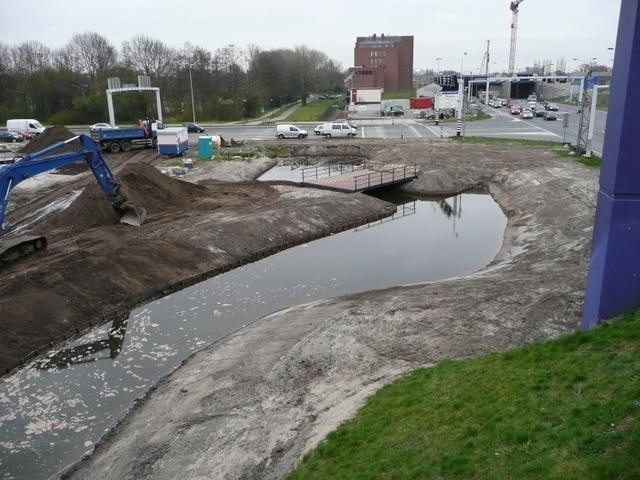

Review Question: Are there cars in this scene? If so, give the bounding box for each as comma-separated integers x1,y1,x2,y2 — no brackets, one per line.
89,123,120,132
183,123,204,133
424,111,450,120
315,119,357,139
276,125,308,139
380,108,404,117
490,95,558,120
0,131,24,143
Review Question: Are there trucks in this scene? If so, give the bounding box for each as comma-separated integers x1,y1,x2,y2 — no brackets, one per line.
7,119,45,137
91,120,167,152
157,126,188,159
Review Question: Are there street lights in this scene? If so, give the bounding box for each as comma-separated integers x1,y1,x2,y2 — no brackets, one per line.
189,63,196,122
573,59,584,74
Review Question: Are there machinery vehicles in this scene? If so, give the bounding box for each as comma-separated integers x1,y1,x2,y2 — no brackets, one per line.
0,135,147,270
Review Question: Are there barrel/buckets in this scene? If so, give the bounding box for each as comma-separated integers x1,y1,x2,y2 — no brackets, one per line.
198,136,212,159
